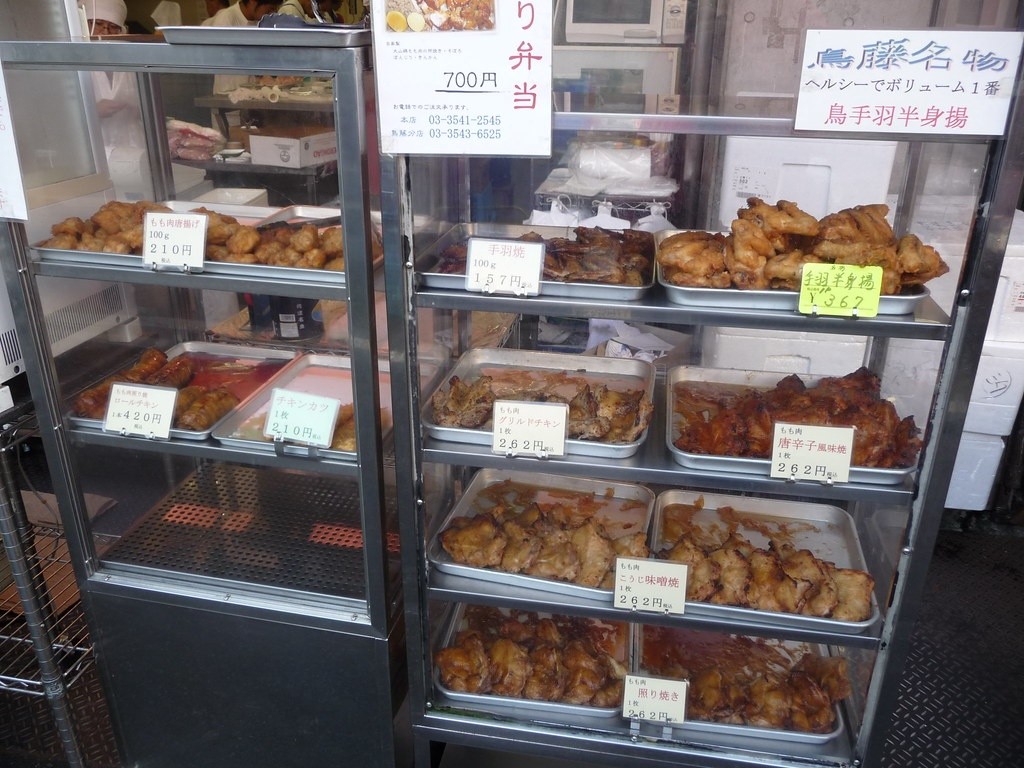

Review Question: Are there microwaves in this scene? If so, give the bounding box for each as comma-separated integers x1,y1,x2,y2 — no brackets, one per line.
565,0,689,45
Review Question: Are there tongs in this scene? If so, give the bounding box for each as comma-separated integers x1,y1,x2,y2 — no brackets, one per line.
258,13,363,30
256,214,342,235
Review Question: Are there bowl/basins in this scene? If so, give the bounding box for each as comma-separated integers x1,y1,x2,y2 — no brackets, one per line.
217,148,245,161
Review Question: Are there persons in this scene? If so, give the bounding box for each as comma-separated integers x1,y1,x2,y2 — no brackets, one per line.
76,0,144,151
200,0,344,126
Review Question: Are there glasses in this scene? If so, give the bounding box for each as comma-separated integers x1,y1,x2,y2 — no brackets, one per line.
90,19,121,34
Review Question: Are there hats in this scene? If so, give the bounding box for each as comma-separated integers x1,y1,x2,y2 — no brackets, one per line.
80,0,127,34
